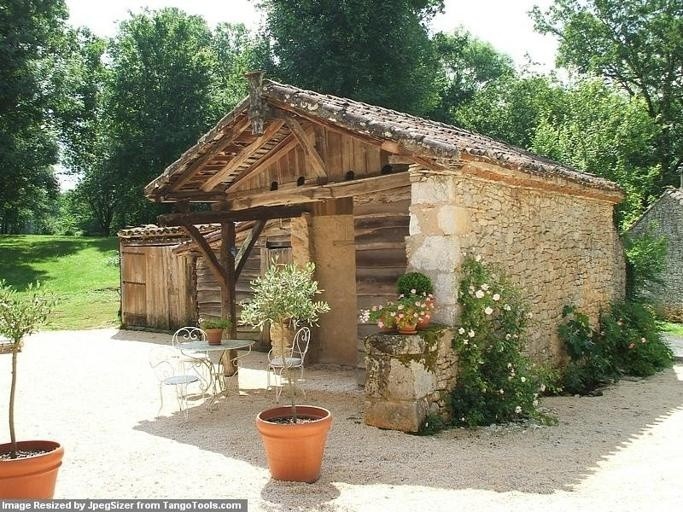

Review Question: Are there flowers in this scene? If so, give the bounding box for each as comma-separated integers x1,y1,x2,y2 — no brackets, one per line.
358,302,396,327
394,290,434,326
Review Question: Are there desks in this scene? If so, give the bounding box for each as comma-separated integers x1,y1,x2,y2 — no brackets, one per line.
176,340,255,413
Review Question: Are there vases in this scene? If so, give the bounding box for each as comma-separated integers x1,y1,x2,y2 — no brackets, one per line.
376,324,398,333
397,320,418,335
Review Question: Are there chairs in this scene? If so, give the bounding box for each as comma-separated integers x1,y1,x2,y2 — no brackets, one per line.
266,326,310,403
148,355,208,422
172,327,212,397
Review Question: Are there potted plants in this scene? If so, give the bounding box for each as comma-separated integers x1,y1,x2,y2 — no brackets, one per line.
0,279,65,501
242,258,333,485
397,271,437,328
200,316,231,345
